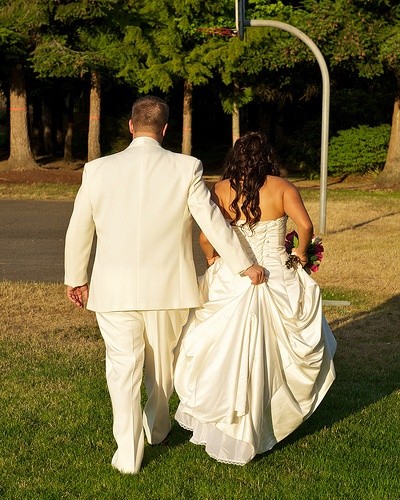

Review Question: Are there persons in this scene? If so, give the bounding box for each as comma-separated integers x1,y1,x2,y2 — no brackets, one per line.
172,132,338,466
64,95,268,475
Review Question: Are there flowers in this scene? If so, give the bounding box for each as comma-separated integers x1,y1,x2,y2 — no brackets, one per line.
284,229,324,275
